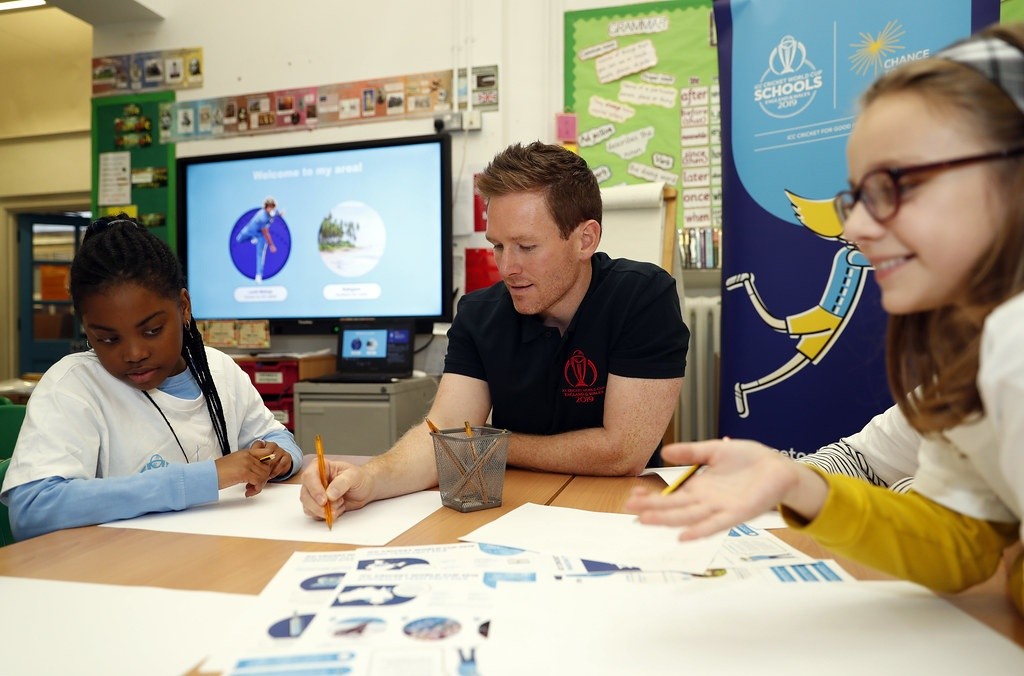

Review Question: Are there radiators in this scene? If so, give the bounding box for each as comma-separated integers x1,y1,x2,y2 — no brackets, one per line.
673,297,722,442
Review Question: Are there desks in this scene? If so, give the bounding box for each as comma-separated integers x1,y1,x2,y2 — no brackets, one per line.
0,451,1024,676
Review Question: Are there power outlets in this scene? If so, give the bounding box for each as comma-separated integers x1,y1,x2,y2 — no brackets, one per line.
433,108,482,133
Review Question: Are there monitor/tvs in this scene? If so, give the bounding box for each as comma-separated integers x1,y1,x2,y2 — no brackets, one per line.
176,131,453,333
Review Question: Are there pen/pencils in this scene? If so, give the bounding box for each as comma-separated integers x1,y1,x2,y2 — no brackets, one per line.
257,452,274,464
313,431,334,532
633,435,732,521
424,416,508,506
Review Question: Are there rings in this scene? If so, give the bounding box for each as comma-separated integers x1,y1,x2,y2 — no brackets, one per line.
250,439,266,448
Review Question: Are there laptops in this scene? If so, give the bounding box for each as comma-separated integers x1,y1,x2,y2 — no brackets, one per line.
301,319,415,383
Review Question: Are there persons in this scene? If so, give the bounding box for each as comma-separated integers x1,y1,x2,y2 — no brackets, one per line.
0,213,303,541
236,196,277,283
300,140,690,522
629,23,1024,619
792,375,1023,649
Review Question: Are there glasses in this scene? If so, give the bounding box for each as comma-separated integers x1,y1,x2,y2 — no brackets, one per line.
833,147,1023,225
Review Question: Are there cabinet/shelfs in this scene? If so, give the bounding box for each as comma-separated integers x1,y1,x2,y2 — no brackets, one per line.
294,373,440,455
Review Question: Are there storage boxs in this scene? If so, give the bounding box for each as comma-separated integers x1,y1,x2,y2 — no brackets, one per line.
231,352,336,434
